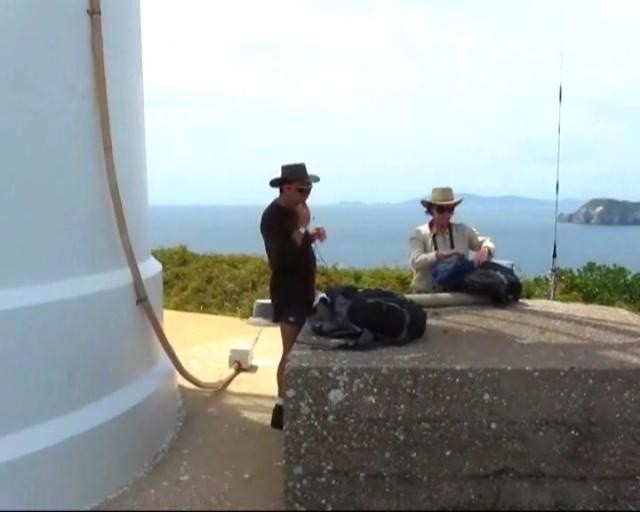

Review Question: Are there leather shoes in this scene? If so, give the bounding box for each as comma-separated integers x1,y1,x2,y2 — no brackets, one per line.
270,404,284,430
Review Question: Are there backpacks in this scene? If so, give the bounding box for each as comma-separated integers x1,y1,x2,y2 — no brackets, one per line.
310,283,427,350
433,259,522,308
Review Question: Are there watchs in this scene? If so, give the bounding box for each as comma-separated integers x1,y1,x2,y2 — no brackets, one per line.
297,223,306,235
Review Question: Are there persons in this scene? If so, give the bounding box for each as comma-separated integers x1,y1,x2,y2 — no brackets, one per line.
408,186,496,294
259,162,326,430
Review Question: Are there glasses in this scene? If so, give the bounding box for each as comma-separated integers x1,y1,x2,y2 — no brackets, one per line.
293,186,313,194
434,204,455,215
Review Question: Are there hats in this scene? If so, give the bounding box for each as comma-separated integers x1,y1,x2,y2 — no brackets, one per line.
421,187,463,208
270,163,320,186
